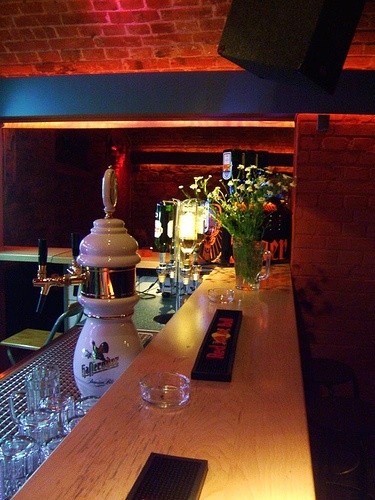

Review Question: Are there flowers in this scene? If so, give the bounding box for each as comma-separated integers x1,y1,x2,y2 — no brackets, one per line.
178,164,297,242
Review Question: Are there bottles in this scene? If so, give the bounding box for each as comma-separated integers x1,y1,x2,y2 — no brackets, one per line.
152,200,208,259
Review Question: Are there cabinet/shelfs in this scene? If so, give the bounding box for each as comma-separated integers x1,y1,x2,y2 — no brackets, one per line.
1,244,315,500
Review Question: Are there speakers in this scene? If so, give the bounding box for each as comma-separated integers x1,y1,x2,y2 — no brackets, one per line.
218,0,365,97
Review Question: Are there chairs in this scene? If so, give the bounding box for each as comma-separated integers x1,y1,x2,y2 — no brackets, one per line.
0,302,86,367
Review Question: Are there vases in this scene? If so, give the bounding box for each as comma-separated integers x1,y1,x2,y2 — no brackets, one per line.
230,235,272,292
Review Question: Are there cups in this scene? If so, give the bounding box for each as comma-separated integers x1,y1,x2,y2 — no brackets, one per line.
157,259,202,295
0,364,101,500
